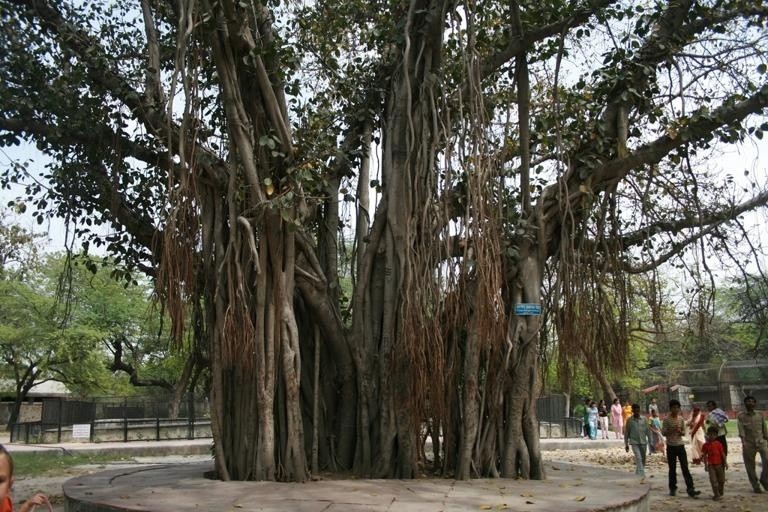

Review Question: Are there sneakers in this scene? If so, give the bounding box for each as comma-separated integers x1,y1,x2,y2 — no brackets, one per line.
688,491,701,497
670,486,678,496
753,487,761,493
760,478,768,491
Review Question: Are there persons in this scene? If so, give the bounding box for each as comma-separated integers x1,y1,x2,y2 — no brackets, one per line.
583,397,664,456
705,401,728,456
661,399,701,497
685,407,705,464
625,404,654,484
0,444,46,512
738,396,768,493
702,427,728,500
